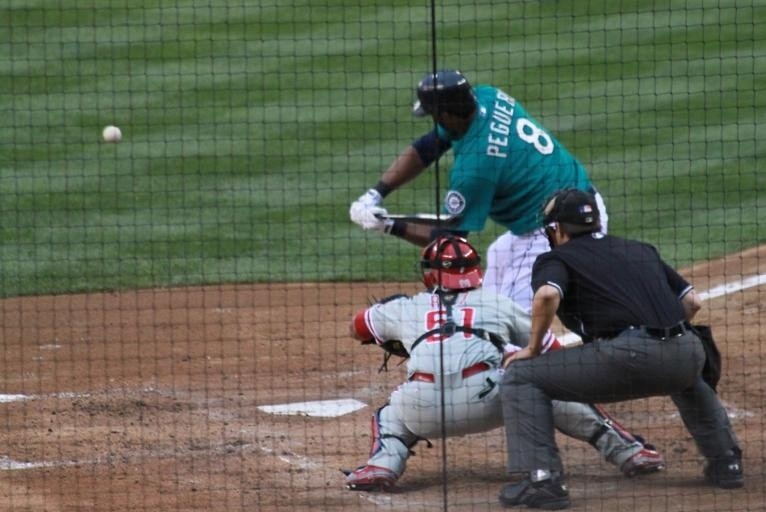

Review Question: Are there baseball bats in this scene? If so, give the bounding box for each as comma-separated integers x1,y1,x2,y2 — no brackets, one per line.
372,213,464,226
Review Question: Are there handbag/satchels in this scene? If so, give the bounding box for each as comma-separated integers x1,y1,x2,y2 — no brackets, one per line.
421,234,483,289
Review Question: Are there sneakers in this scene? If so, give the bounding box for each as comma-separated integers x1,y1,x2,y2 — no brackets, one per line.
705,458,744,487
498,474,569,510
344,465,398,491
620,448,666,477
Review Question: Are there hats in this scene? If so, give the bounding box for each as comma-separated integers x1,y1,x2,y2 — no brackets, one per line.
412,69,470,117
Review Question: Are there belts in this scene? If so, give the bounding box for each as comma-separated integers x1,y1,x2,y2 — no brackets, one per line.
646,320,689,338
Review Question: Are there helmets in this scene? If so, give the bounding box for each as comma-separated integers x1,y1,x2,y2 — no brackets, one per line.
543,188,601,235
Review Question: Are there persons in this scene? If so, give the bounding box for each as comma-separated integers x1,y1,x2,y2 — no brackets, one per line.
349,69,609,316
345,235,664,490
498,189,744,510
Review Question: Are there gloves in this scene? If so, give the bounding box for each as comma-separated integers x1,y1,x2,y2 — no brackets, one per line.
349,181,407,238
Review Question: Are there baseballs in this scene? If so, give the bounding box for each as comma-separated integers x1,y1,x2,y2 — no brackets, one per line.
103,125,123,144
